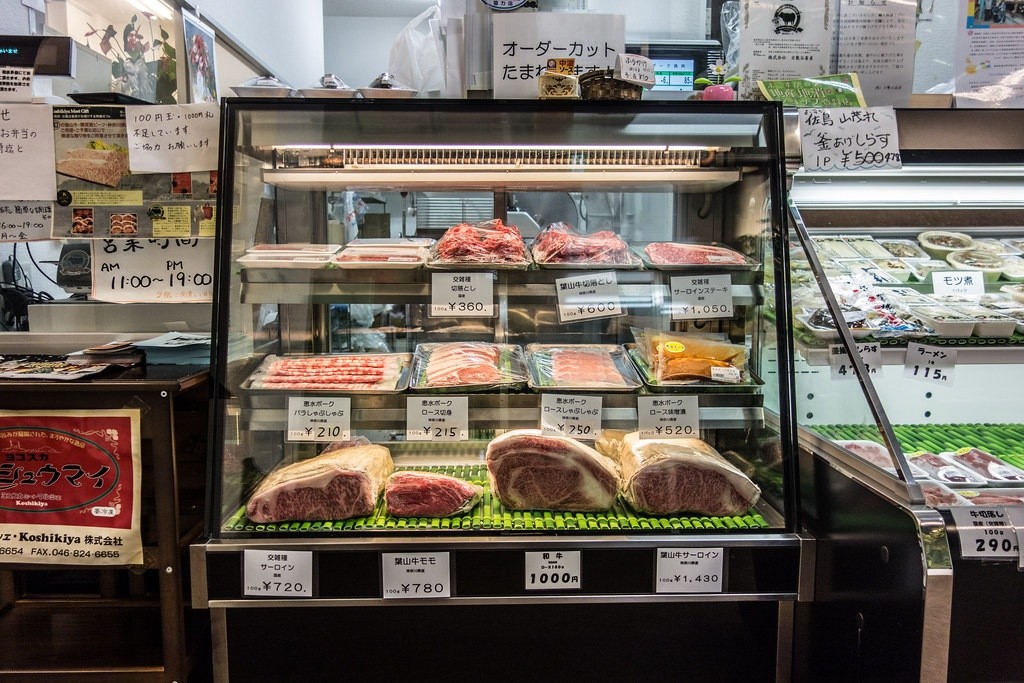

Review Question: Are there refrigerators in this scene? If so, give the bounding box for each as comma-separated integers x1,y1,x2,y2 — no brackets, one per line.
766,106,1024,683
186,97,816,683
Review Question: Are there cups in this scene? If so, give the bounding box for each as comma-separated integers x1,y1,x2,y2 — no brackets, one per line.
464,0,491,90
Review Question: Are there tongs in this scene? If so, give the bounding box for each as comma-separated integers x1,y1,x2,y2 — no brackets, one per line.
407,192,417,218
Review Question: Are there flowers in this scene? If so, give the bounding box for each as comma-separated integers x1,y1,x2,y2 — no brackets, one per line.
85,9,178,105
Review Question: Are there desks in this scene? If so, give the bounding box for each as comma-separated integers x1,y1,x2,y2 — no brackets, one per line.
0,352,286,683
